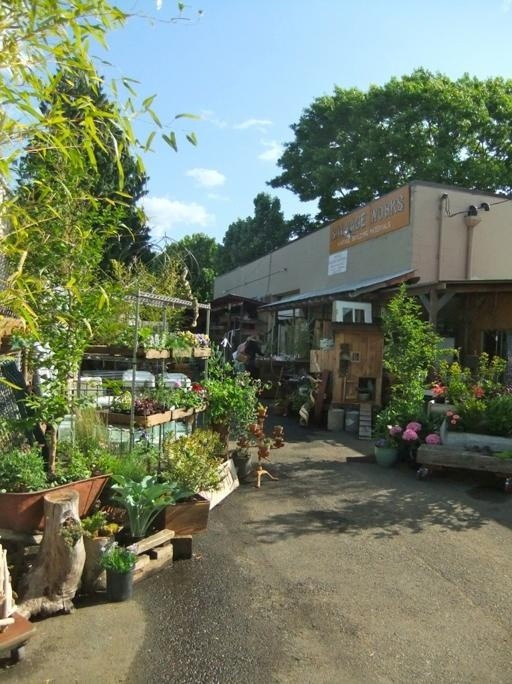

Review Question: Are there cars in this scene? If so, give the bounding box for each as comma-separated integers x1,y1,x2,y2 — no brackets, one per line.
155,372,191,388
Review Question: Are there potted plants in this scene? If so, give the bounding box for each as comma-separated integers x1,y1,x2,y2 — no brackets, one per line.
371,409,401,468
1,251,229,604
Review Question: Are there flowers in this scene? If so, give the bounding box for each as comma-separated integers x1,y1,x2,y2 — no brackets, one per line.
387,418,442,459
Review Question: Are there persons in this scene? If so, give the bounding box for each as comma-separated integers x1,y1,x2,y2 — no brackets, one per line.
242,334,267,372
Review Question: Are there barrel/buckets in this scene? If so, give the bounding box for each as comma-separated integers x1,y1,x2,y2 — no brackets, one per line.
344,411,359,433
327,408,344,433
